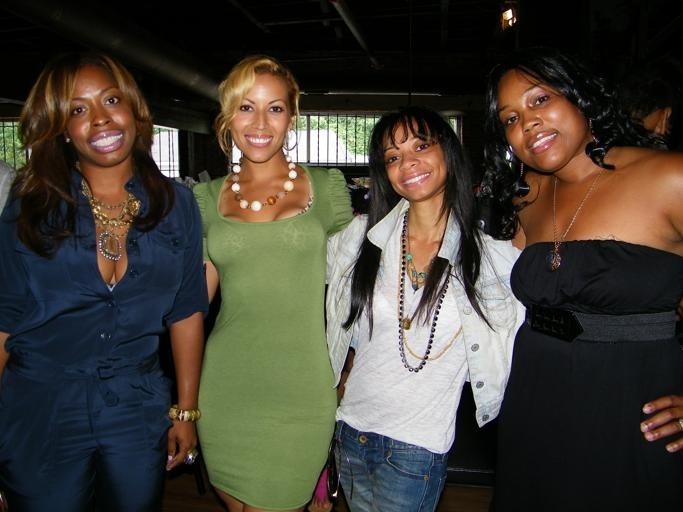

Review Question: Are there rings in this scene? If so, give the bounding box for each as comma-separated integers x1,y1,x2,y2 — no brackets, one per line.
678,417,682,429
184,447,198,464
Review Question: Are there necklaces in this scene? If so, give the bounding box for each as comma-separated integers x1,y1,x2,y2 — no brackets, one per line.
77,164,140,263
230,152,298,212
397,207,463,373
543,167,606,272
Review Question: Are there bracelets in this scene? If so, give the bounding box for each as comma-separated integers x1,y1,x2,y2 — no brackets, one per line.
168,403,200,422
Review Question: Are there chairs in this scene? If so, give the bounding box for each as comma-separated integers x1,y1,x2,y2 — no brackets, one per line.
444,409,513,486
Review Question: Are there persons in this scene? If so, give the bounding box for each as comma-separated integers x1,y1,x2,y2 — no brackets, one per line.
189,50,355,511
324,97,528,512
474,41,682,508
0,45,211,512
